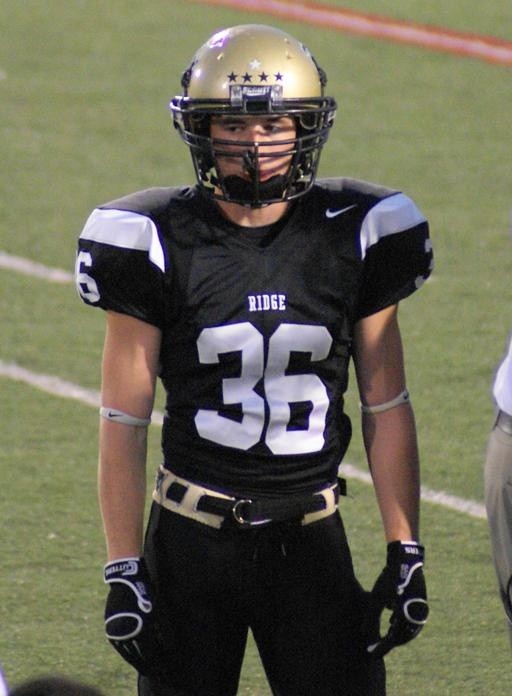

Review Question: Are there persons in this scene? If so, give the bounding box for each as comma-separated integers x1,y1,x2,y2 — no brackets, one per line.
74,23,439,695
481,334,512,635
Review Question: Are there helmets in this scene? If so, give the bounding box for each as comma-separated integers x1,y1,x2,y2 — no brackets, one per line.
181,24,327,121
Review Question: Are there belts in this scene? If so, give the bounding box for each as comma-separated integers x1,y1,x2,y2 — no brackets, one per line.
166,482,339,524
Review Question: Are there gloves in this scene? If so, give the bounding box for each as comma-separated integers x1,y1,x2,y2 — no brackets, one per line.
367,540,429,655
104,557,158,676
